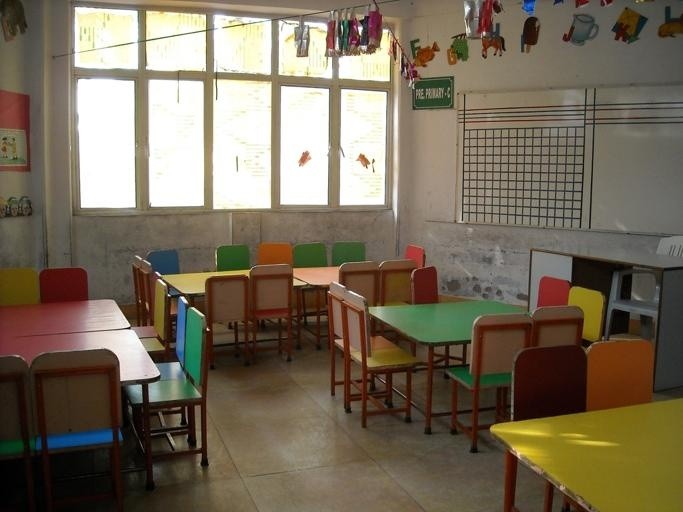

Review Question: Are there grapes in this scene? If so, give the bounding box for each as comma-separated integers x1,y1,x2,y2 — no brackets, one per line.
452,36,469,61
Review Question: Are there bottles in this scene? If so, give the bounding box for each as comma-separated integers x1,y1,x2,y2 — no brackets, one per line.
0,195,32,218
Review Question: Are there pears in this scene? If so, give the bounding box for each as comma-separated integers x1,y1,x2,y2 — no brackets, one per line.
447,48,457,65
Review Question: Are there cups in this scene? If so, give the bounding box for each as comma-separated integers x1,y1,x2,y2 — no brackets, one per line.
568,14,598,46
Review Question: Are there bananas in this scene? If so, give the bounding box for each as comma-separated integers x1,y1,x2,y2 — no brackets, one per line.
658,18,683,37
